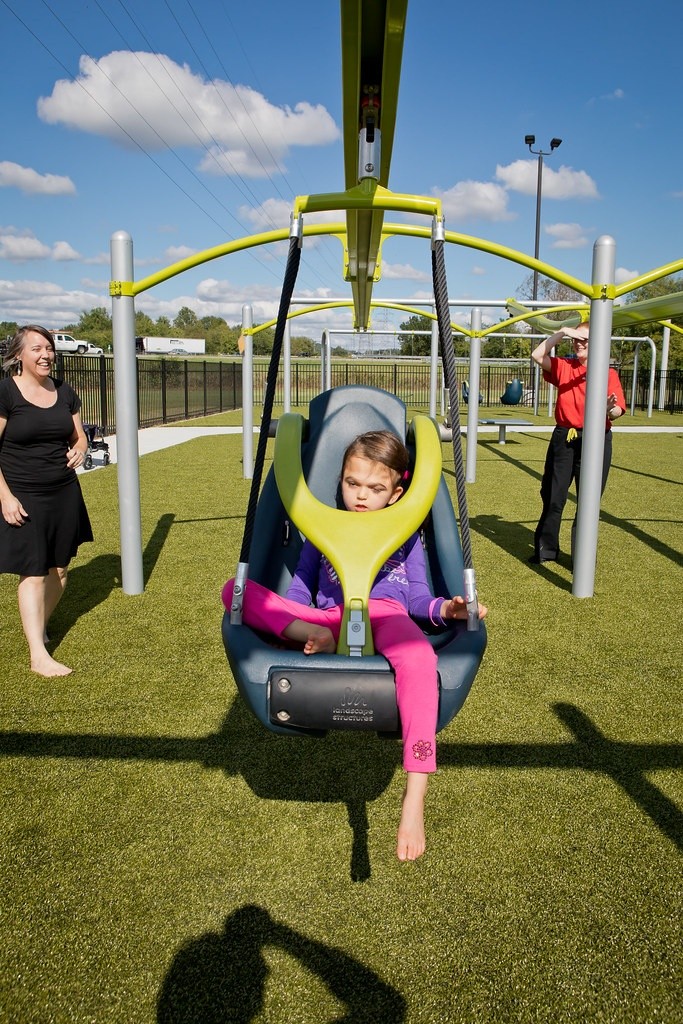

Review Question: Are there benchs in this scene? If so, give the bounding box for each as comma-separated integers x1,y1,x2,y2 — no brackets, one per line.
478,418,534,444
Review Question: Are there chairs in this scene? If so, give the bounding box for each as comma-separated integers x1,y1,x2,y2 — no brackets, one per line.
500,379,522,405
221,383,490,740
461,381,484,405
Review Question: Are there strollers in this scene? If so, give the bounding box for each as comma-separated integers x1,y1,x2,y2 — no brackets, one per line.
81,424,109,470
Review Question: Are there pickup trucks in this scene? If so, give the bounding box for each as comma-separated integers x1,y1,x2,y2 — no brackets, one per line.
51,333,88,354
85,343,103,354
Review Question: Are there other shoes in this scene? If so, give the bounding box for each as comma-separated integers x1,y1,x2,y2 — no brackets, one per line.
529,556,554,564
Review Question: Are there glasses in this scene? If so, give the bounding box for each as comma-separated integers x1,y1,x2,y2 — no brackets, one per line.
572,338,590,344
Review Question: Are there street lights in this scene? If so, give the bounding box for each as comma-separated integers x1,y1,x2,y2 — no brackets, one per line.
525,134,562,392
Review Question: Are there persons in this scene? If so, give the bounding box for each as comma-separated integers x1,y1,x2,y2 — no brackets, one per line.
0,324,95,679
529,320,627,573
222,428,488,861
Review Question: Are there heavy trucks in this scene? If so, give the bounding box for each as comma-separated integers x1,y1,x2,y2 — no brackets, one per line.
135,336,207,355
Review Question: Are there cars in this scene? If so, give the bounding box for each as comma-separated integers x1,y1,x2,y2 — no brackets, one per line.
168,348,188,355
